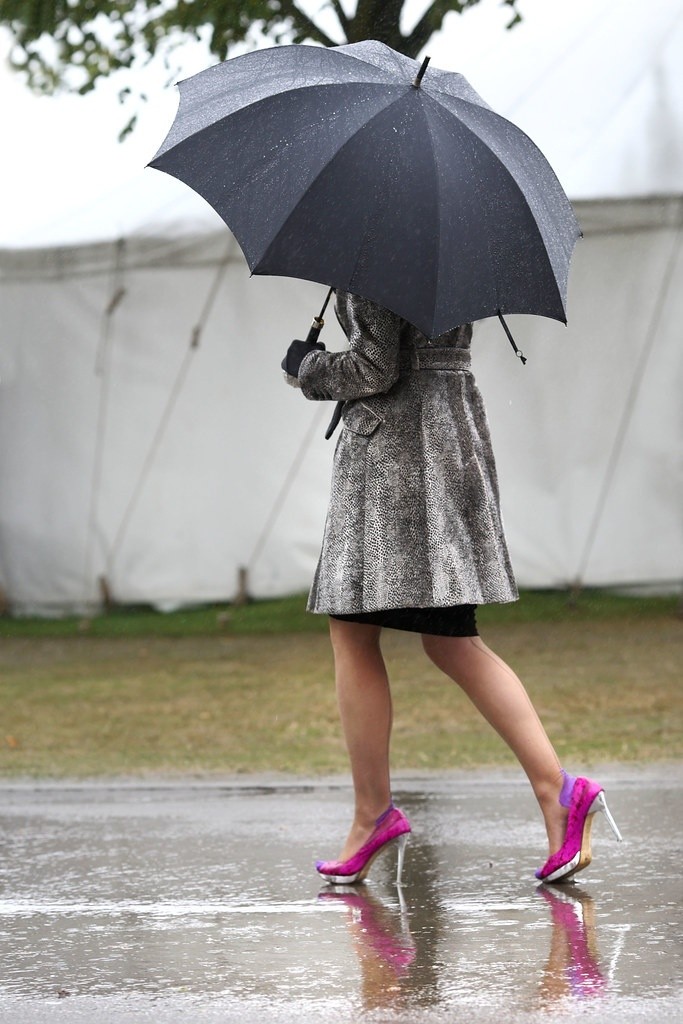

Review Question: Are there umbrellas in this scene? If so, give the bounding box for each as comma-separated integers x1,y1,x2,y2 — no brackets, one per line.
143,38,581,387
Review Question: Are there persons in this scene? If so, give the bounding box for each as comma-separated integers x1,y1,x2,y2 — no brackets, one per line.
279,282,622,891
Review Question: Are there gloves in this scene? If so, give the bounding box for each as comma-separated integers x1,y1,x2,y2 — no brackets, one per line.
281,339,326,376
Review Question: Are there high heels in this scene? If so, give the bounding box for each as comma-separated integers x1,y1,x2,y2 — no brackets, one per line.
534,883,628,997
316,807,412,887
317,887,413,970
535,778,624,883
